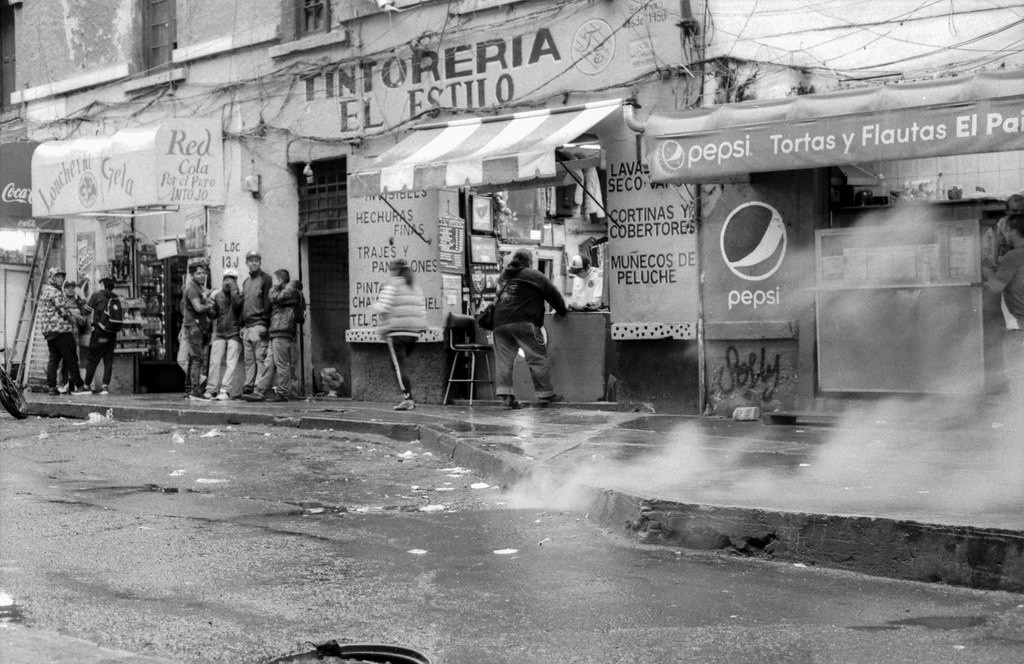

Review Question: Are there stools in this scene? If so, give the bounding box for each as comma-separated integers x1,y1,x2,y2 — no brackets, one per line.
443,312,494,407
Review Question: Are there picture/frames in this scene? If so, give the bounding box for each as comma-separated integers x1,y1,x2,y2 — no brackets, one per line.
470,234,498,265
472,196,494,231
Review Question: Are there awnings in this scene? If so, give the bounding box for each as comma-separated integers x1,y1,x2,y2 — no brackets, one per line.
347,98,626,243
0,143,64,234
645,68,1024,186
32,118,225,218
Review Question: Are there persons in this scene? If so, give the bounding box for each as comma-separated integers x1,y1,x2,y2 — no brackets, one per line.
238,252,273,401
184,262,220,401
566,255,602,311
189,269,243,401
269,269,299,401
70,278,117,395
60,281,87,387
981,194,1024,330
39,266,84,395
492,249,567,407
370,258,425,410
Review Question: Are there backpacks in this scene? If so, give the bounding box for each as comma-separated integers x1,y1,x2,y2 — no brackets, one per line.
95,290,123,332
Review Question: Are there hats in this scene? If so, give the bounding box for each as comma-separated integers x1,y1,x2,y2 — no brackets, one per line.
568,254,590,273
64,280,76,287
246,250,262,259
223,269,238,279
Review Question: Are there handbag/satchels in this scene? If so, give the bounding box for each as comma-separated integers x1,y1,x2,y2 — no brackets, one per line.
477,303,494,331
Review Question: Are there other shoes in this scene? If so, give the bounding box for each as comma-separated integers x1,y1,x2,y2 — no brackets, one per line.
539,394,564,403
505,395,523,407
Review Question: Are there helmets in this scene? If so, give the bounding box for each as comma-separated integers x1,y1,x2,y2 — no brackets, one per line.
48,267,67,284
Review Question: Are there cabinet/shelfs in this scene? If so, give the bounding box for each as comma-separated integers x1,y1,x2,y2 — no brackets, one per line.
139,256,187,393
112,303,149,354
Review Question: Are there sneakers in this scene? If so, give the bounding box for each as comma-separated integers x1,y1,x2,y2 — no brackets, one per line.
100,386,109,394
242,393,264,402
71,387,93,395
49,387,61,396
393,399,414,410
189,392,211,401
215,392,229,400
266,393,287,402
